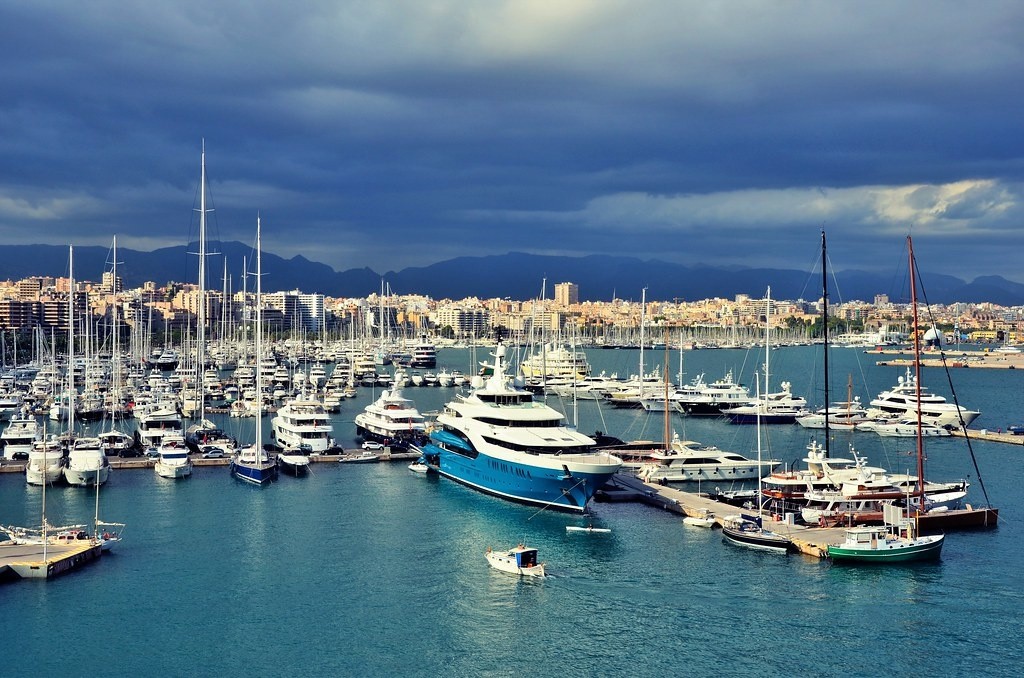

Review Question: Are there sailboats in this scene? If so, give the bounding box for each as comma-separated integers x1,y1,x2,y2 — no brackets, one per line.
0,422,126,552
0,138,1024,530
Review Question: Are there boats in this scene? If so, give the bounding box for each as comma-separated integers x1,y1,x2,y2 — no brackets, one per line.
682,515,716,528
826,469,945,563
408,461,429,473
721,512,792,552
483,531,548,579
